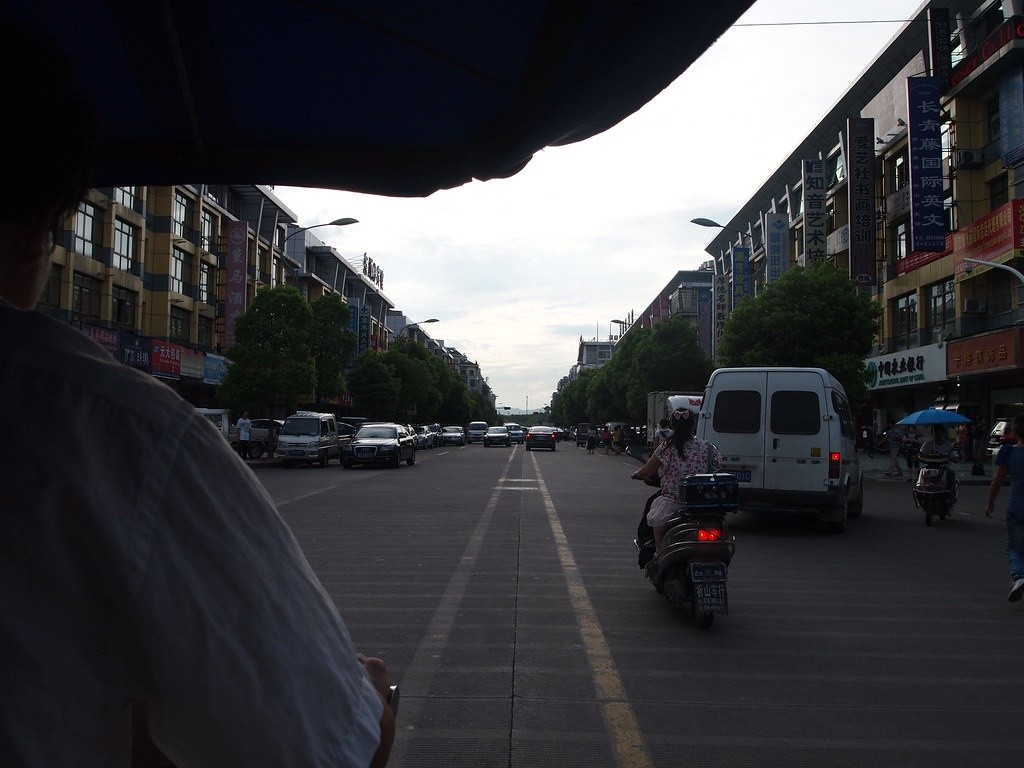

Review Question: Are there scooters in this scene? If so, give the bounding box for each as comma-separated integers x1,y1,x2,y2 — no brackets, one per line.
625,443,740,631
863,430,892,459
911,448,961,526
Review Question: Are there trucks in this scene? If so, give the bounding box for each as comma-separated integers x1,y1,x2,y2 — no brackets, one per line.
274,413,351,467
192,408,278,458
646,391,703,459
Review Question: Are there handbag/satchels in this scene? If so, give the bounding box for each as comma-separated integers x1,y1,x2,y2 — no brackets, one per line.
705,440,719,474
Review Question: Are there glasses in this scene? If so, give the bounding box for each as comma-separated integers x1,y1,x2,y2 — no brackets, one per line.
46,227,58,255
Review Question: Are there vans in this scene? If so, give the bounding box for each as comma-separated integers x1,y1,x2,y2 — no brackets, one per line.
696,367,863,535
575,423,599,447
466,421,489,444
606,422,630,438
502,422,524,445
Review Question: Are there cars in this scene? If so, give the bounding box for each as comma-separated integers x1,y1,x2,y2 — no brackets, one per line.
553,425,604,442
525,426,555,452
239,418,290,443
337,422,465,470
482,426,512,448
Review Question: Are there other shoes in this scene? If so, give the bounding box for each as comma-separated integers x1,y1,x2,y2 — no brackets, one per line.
907,478,913,481
885,473,892,477
894,472,903,476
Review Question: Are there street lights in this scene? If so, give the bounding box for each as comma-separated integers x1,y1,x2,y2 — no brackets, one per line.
280,218,358,288
691,218,766,306
398,319,439,340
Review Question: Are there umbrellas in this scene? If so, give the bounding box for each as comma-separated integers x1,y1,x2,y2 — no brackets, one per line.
895,407,975,453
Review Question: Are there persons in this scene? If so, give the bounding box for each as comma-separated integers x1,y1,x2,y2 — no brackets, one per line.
984,413,1024,602
587,424,624,455
634,407,722,558
655,418,673,445
957,423,970,463
919,423,962,490
235,407,253,462
0,14,396,768
887,420,904,479
902,425,921,482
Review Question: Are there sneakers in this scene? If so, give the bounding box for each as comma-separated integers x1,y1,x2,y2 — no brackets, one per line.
1008,578,1024,602
